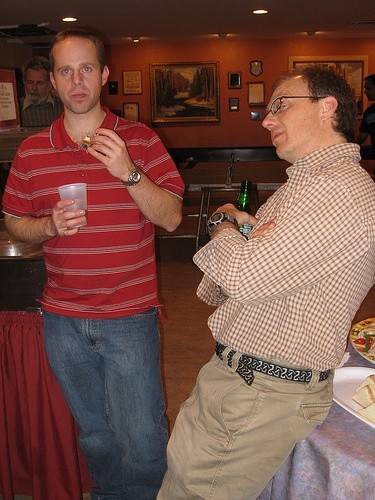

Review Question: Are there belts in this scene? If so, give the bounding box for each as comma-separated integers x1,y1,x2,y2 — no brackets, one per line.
215,341,331,386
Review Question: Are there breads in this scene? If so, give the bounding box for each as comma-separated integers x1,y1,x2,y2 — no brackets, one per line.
353,374,375,423
80,136,102,144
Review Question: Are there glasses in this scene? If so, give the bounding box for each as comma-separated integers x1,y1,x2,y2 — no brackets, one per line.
261,96,328,131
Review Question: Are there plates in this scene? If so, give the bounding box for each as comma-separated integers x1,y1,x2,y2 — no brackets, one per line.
332,366,375,429
349,317,375,364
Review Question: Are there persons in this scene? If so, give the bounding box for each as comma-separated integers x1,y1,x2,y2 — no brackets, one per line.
154,63,375,500
1,29,185,500
20,56,64,127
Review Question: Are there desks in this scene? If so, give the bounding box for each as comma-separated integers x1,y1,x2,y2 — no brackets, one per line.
181,160,289,256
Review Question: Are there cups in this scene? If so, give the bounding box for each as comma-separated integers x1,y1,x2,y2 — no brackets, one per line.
57,183,88,228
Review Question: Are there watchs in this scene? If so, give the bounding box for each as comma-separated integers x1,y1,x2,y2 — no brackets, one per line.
120,166,141,186
207,212,239,241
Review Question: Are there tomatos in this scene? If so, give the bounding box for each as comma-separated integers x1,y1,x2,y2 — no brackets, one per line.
354,337,373,352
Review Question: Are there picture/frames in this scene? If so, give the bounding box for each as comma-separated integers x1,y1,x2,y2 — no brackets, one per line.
229,97,239,112
288,55,369,119
148,61,221,127
228,71,242,89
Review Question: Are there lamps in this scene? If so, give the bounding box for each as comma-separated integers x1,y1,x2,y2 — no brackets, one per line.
307,31,316,37
217,34,227,39
131,37,140,43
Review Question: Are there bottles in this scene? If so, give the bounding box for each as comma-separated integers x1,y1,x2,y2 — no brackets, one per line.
234,180,252,240
